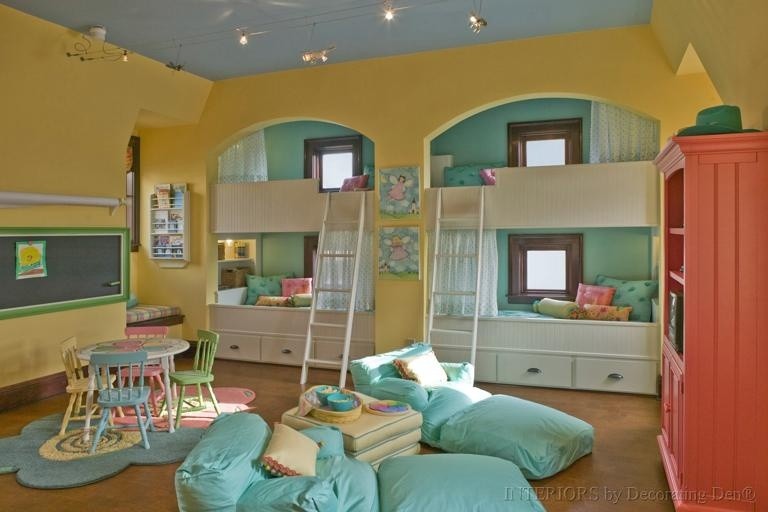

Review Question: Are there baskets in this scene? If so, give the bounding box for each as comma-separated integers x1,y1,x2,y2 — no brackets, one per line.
304,384,364,423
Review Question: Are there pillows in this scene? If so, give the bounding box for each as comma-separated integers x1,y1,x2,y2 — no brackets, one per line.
246,269,312,307
392,350,450,389
258,419,321,477
479,166,508,185
339,174,370,192
532,274,655,321
363,165,373,189
444,161,505,187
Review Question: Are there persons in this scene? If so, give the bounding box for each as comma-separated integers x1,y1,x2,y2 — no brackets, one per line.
383,173,414,205
384,234,411,261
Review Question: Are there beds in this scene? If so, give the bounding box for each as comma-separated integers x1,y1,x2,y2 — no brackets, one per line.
425,161,660,397
173,410,547,512
209,178,376,372
348,340,596,482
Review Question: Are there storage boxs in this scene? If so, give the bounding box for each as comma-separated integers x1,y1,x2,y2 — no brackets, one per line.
430,153,456,187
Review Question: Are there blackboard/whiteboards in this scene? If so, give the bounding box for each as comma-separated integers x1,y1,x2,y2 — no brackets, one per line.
0,237,121,312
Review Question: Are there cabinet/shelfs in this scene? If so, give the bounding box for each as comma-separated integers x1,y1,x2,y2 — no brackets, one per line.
146,181,192,270
218,240,256,290
651,132,768,512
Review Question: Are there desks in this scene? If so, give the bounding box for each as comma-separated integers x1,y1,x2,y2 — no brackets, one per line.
75,337,193,441
279,391,425,474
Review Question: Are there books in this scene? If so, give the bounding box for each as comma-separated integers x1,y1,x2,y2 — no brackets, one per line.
152,182,187,259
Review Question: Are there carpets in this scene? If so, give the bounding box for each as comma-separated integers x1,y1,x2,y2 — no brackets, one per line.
0,384,258,491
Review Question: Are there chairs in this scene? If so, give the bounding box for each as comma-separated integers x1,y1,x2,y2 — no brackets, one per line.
111,326,169,416
56,335,125,437
157,328,227,430
88,352,155,453
